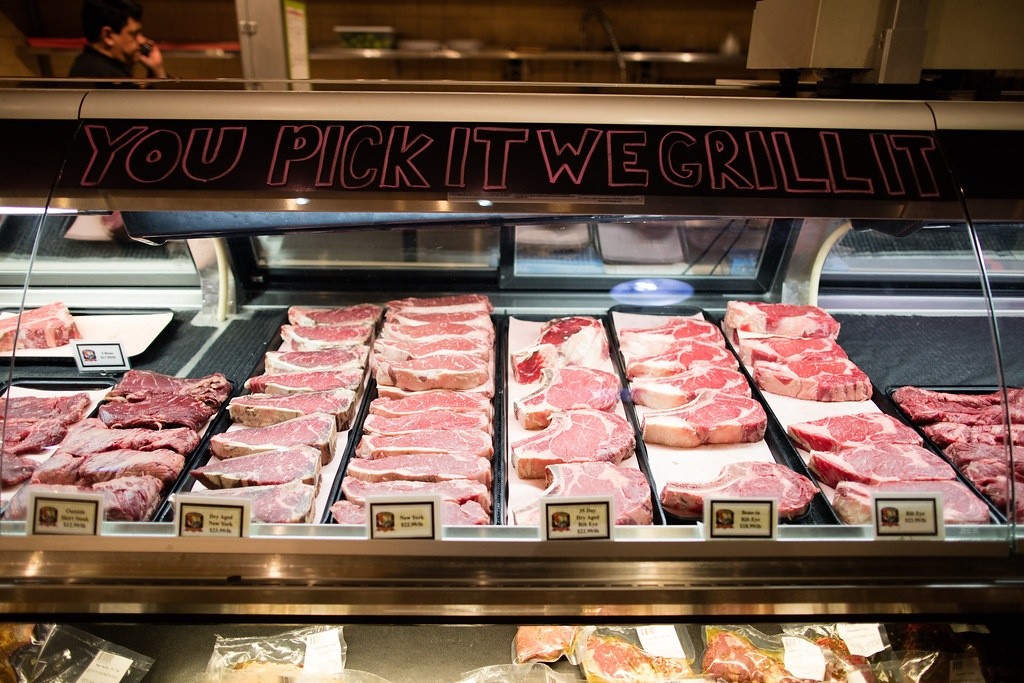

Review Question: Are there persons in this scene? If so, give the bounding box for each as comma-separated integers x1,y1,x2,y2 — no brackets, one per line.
68,0,179,89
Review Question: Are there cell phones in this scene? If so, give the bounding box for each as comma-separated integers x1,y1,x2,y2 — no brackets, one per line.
141,45,152,56
